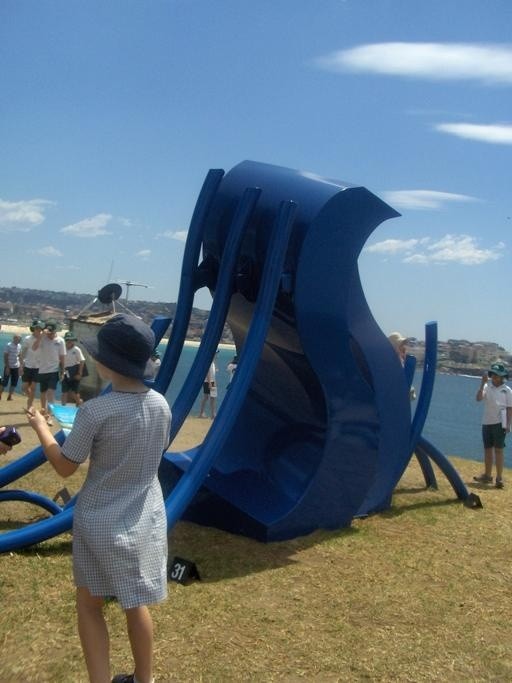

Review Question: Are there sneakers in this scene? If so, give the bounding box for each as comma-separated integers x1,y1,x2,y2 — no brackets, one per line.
496,478,504,488
473,473,492,484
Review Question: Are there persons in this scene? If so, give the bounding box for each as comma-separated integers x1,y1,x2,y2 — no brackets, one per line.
0,335,22,401
385,332,417,402
27,314,176,683
151,348,163,382
61,332,85,407
199,350,220,421
224,354,239,391
473,363,511,487
0,426,13,456
19,321,44,410
32,321,67,415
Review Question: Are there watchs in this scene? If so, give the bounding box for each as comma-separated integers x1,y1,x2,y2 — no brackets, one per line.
60,371,66,375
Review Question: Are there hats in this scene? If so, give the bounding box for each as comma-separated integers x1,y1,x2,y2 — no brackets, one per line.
388,332,417,346
79,314,156,379
45,321,57,333
30,320,43,332
64,332,78,342
487,364,507,378
